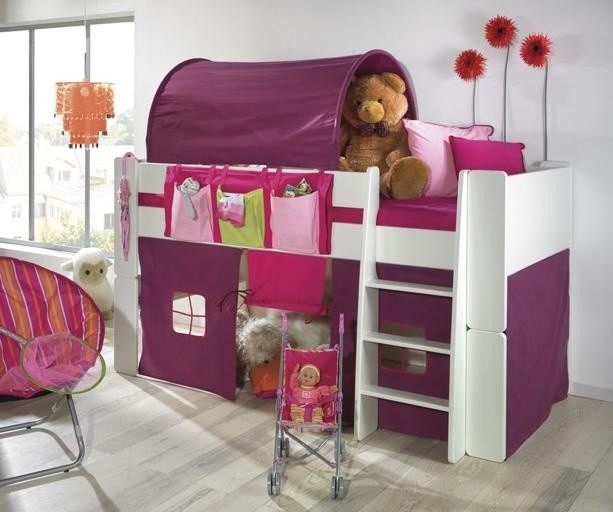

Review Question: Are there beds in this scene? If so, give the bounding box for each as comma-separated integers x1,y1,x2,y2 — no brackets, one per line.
112,153,574,464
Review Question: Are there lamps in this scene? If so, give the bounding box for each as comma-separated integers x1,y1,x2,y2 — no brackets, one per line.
51,1,115,151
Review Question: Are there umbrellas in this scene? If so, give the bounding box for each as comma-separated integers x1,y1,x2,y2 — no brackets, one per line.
118,151,134,262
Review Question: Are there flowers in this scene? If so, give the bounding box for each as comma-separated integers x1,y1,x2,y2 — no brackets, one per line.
454,14,551,165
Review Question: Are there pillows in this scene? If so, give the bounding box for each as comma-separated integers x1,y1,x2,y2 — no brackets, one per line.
402,117,527,200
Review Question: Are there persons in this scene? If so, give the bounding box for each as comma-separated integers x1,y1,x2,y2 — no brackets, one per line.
290,363,339,423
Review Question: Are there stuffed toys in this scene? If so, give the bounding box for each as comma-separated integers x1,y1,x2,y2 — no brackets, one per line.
61,247,114,320
339,72,429,200
